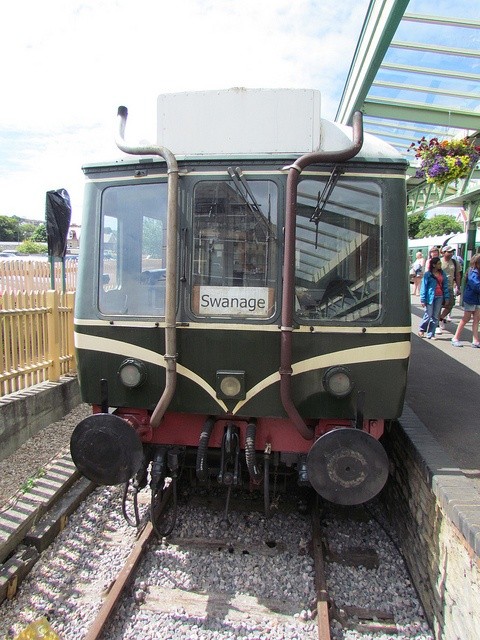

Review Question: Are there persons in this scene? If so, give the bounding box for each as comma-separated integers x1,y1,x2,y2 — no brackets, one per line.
446,252,464,322
435,246,460,335
424,247,440,272
411,251,427,296
419,259,448,339
451,253,480,348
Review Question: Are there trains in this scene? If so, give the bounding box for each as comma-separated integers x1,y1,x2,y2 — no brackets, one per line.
68,77,414,542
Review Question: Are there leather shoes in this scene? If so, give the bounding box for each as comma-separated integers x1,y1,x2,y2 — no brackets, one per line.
418,332,426,338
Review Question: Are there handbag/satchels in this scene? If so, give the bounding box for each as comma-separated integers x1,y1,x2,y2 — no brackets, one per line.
442,294,445,305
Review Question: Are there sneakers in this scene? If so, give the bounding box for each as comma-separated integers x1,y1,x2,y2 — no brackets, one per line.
451,339,463,347
472,343,480,348
446,315,451,322
435,327,442,335
439,320,445,330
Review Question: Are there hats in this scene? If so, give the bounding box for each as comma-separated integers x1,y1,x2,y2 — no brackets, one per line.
442,246,456,252
